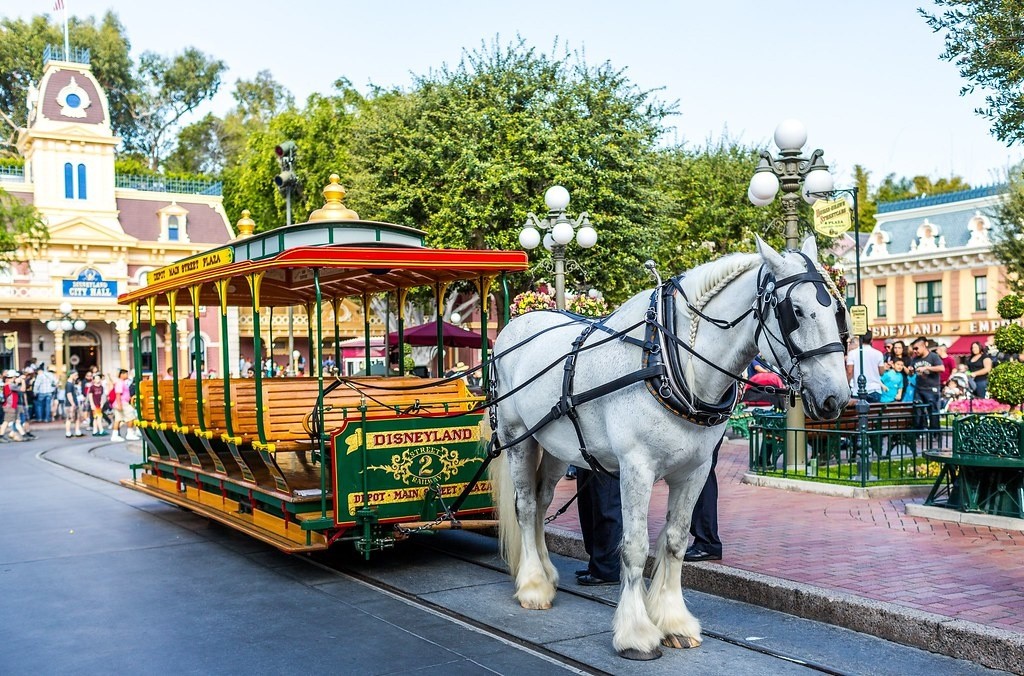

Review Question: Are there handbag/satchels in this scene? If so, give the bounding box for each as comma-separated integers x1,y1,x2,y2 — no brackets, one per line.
106,389,120,404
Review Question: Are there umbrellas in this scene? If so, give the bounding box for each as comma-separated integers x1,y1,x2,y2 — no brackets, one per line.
383,318,493,349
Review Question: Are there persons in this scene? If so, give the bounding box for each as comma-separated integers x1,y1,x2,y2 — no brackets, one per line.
747,336,1024,461
164,352,342,380
564,434,722,587
65,365,141,442
0,359,61,441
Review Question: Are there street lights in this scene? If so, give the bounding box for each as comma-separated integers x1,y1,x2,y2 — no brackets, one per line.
519,184,598,310
46,302,87,380
748,117,839,470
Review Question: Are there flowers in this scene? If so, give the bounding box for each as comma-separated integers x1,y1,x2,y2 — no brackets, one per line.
563,289,611,318
509,290,556,322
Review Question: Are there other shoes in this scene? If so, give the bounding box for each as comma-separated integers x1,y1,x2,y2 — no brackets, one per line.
111,436,125,442
86,427,94,430
66,433,75,439
93,430,109,437
76,433,88,439
124,432,139,440
0,430,36,442
108,424,113,429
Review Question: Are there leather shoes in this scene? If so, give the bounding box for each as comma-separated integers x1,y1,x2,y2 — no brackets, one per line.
577,574,618,586
684,548,720,561
574,568,592,576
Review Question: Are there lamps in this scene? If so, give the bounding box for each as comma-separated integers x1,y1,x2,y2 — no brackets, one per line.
39,335,44,351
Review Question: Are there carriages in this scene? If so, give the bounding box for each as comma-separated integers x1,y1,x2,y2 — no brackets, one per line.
117,218,853,663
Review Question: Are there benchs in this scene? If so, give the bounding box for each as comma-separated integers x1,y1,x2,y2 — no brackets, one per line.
765,402,920,467
138,373,467,454
921,413,1024,519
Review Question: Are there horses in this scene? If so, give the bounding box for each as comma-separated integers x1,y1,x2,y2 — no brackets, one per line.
476,232,851,662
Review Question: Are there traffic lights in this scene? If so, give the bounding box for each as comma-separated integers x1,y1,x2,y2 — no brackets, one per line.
274,141,295,189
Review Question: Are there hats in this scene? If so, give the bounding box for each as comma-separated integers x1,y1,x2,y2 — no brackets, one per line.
884,338,894,343
248,366,256,374
7,369,20,377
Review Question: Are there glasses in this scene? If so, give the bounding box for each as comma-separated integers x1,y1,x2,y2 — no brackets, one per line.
912,344,923,351
884,344,892,349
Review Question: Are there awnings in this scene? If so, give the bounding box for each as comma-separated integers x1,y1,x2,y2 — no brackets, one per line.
946,336,988,355
